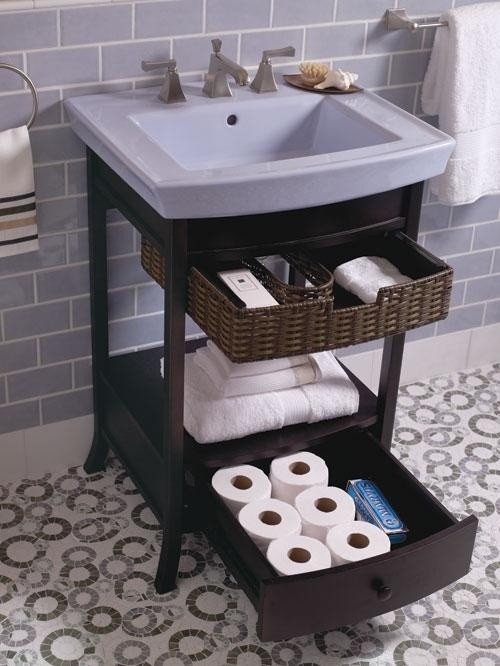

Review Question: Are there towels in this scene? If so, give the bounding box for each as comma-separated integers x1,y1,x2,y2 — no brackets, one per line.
0,126,40,260
334,251,414,307
158,339,360,446
419,1,500,206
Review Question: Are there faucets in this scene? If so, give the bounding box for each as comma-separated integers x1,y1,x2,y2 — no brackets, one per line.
203,40,251,99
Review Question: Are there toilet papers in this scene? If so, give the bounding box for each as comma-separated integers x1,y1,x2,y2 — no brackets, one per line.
214,450,396,576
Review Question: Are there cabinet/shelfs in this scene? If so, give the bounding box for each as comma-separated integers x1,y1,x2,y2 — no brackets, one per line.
75,155,478,652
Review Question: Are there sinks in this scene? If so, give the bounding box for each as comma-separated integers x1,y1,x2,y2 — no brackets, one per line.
64,74,456,220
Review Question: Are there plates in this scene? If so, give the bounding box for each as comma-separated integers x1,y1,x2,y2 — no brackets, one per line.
282,72,364,95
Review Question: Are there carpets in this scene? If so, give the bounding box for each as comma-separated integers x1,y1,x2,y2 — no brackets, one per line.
3,367,498,666
